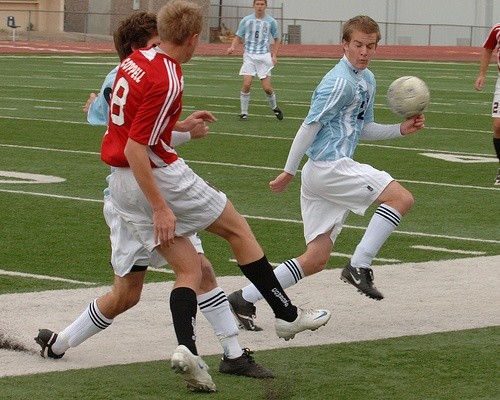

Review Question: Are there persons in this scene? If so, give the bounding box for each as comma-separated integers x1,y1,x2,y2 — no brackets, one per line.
34,12,275,378
227,0,283,120
227,15,426,331
101,2,331,394
475,23,500,184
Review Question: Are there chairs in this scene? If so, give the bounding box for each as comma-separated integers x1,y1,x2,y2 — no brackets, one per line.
7,16,21,40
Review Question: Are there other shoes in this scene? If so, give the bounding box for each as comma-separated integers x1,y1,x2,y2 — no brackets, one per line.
493,176,500,185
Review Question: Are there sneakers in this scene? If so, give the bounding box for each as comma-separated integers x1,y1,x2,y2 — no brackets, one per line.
219,348,275,378
170,345,216,392
275,305,331,341
34,329,65,359
241,114,248,119
227,289,262,331
340,261,383,300
271,106,284,120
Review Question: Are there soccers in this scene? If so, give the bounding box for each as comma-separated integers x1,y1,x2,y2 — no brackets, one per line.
387,76,430,119
